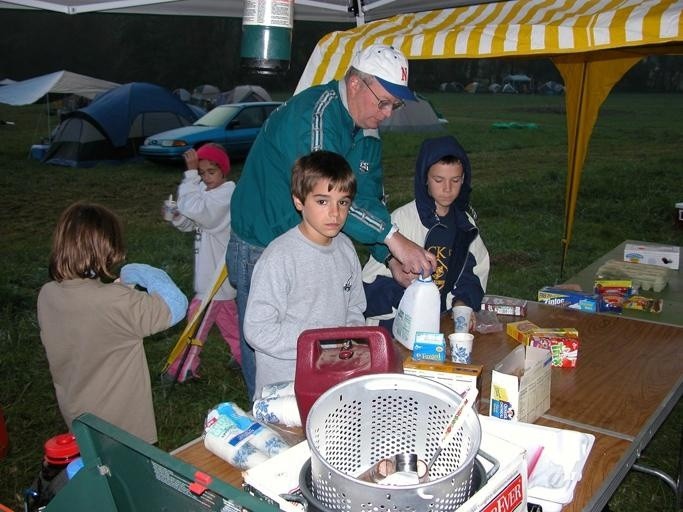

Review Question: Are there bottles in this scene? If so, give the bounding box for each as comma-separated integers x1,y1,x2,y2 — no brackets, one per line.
37,431,79,494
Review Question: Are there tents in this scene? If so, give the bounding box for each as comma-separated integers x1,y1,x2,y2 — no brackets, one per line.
375,89,448,129
431,77,563,96
174,83,275,107
29,79,198,169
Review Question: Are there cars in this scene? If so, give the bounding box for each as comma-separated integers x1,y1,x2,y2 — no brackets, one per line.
140,101,284,164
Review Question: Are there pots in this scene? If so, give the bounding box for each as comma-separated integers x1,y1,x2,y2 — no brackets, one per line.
295,446,498,512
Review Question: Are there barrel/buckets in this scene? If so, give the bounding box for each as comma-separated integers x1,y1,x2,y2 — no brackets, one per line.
295,327,405,437
392,272,441,352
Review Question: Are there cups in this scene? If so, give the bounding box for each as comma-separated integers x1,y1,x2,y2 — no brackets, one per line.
452,305,472,334
162,200,177,221
448,333,474,363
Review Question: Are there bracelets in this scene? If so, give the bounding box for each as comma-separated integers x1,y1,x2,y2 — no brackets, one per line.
381,253,394,270
385,221,397,239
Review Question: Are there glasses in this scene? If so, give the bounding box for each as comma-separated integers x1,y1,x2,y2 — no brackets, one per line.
360,78,407,113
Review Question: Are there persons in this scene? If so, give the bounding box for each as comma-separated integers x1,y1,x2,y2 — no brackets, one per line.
224,45,437,393
356,134,490,336
238,148,370,397
160,139,243,385
35,201,189,451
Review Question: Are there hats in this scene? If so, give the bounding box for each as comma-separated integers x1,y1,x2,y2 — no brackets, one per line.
350,44,421,103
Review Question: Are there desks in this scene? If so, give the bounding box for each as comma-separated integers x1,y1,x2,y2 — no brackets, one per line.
555,239,682,328
168,301,683,512
